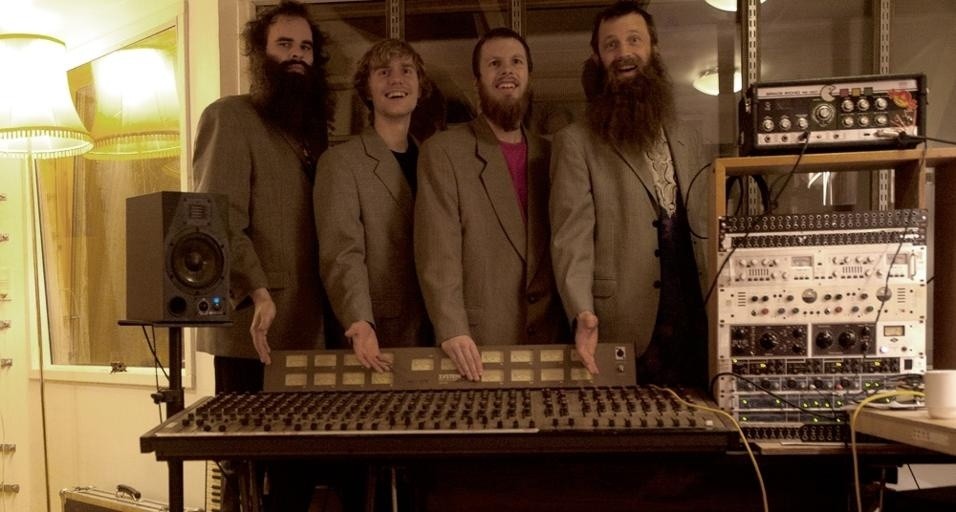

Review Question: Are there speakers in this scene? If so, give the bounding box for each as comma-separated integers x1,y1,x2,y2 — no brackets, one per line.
126,191,231,323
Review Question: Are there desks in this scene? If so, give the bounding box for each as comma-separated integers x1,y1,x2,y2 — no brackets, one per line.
849,404,956,458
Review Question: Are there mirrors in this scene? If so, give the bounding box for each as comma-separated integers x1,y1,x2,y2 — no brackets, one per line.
36,26,189,372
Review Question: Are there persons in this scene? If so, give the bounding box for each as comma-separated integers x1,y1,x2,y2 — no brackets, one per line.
413,27,552,383
192,0,332,395
548,4,713,375
313,39,435,375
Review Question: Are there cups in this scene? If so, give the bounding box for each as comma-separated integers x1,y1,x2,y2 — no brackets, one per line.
923,368,956,419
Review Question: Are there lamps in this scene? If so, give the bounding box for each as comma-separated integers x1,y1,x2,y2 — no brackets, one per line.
90,47,183,163
0,32,94,160
